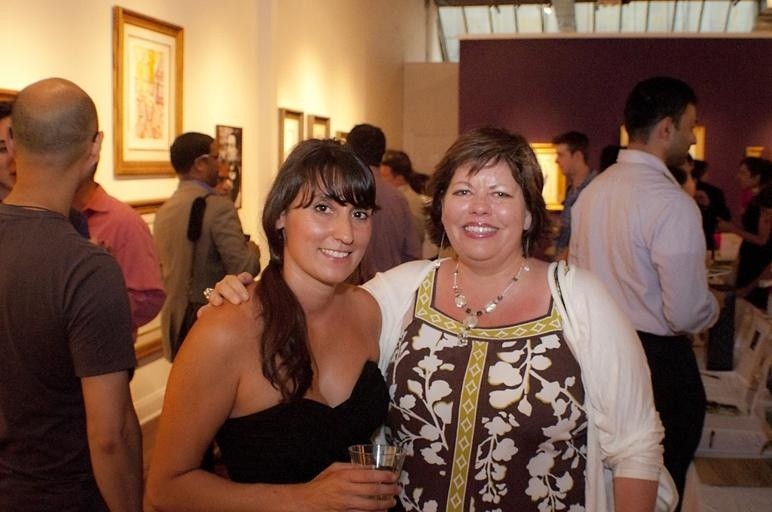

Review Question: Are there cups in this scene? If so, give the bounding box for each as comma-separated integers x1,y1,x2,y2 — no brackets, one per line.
346,443,409,506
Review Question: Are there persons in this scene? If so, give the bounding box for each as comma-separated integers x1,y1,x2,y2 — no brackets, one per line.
567,75,722,512
203,124,668,512
552,131,772,314
0,101,167,345
143,136,403,512
344,124,441,288
155,132,261,363
0,75,146,512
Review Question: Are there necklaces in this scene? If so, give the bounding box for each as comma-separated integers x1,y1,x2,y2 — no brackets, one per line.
452,255,531,331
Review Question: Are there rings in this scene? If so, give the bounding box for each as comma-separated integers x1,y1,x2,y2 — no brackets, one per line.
203,287,214,299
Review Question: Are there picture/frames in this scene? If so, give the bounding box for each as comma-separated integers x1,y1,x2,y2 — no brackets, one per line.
619,124,704,161
120,197,170,371
532,142,566,211
307,115,331,140
112,4,187,182
278,107,305,169
